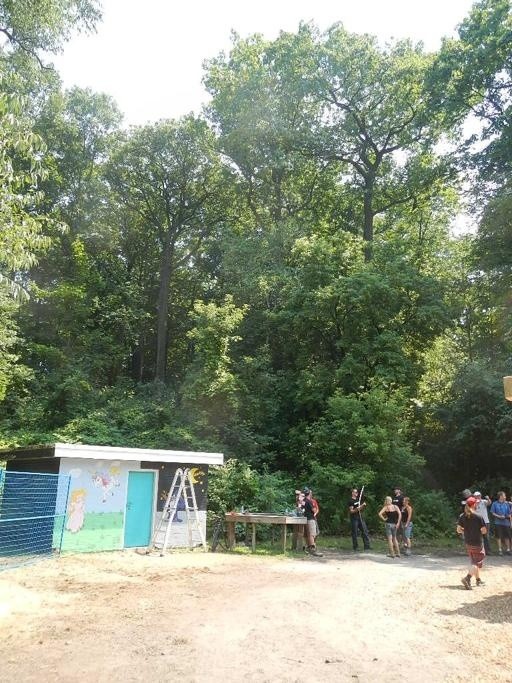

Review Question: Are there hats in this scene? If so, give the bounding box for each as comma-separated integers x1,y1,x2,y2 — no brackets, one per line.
462,488,482,506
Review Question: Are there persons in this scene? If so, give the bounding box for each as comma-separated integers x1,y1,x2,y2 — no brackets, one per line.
349,488,373,550
294,487,323,556
392,487,408,547
399,497,413,556
379,496,402,558
456,489,512,590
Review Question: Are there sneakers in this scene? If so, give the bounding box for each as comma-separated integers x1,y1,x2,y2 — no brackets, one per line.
506,550,512,554
462,576,472,589
497,551,504,555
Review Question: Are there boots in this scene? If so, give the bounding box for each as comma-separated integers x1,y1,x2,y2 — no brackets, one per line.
394,549,400,556
387,550,395,557
